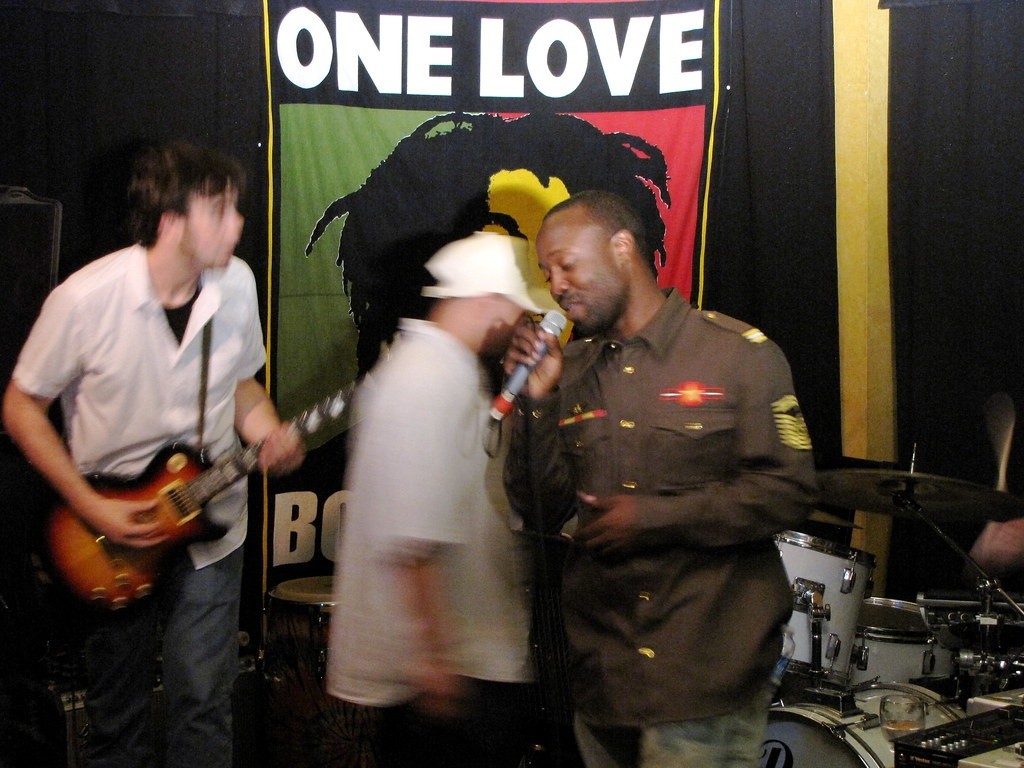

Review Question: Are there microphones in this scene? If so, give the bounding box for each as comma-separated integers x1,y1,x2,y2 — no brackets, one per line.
489,311,567,421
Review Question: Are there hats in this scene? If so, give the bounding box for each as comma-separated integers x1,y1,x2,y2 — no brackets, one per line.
422,231,543,315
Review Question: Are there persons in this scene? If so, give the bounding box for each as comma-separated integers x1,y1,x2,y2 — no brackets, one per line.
790,579,825,614
3,144,306,768
506,191,821,768
327,231,545,768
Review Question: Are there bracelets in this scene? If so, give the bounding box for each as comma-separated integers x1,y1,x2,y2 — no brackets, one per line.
428,641,465,665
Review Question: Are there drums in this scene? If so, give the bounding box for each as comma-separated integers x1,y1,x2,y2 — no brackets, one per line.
772,528,881,688
846,594,960,686
758,680,969,768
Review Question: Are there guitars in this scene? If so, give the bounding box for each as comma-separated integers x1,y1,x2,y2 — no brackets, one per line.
29,373,367,628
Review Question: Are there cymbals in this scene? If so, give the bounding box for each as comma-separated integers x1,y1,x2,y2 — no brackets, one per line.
817,467,1024,527
